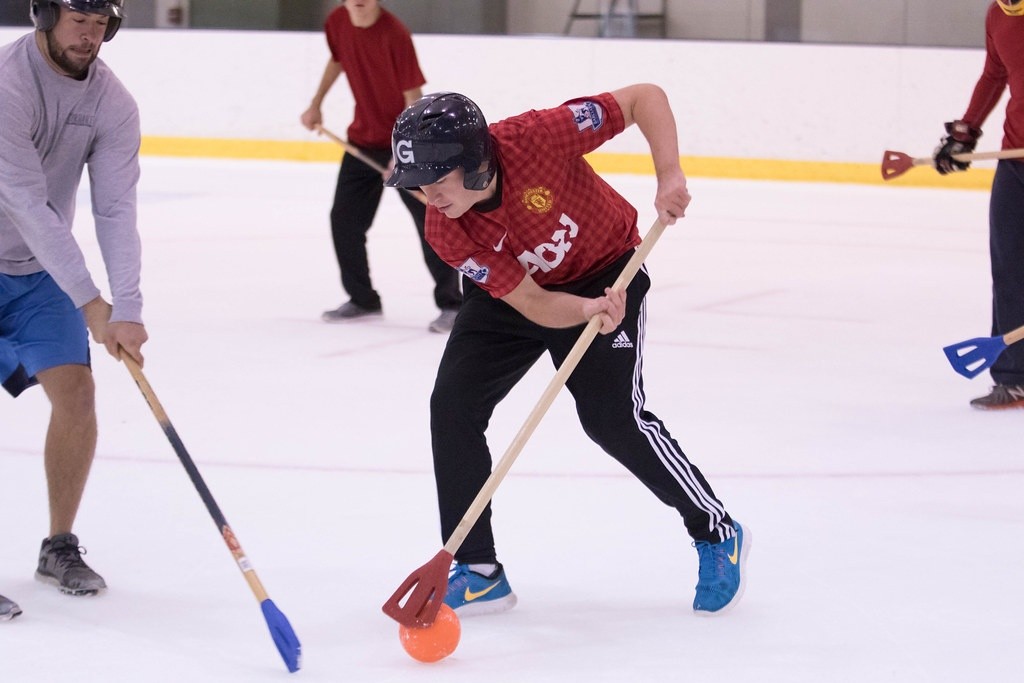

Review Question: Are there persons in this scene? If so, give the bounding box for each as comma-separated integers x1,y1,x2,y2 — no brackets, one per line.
300,0,463,333
934,0,1024,411
383,83,754,616
0,0,149,626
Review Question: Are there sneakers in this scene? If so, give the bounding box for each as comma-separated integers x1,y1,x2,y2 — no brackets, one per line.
693,522,750,616
431,306,457,333
0,592,24,621
970,382,1024,408
443,564,517,618
27,0,123,43
932,124,983,176
34,530,106,594
321,296,383,323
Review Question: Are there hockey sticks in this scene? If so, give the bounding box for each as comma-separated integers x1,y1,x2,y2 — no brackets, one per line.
116,346,302,675
880,147,1022,181
940,325,1022,382
381,215,668,630
313,123,427,204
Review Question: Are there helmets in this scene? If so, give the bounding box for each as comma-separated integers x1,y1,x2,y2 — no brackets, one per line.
383,93,498,190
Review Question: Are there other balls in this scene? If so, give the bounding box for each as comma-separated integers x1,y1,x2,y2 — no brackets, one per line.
395,600,461,664
